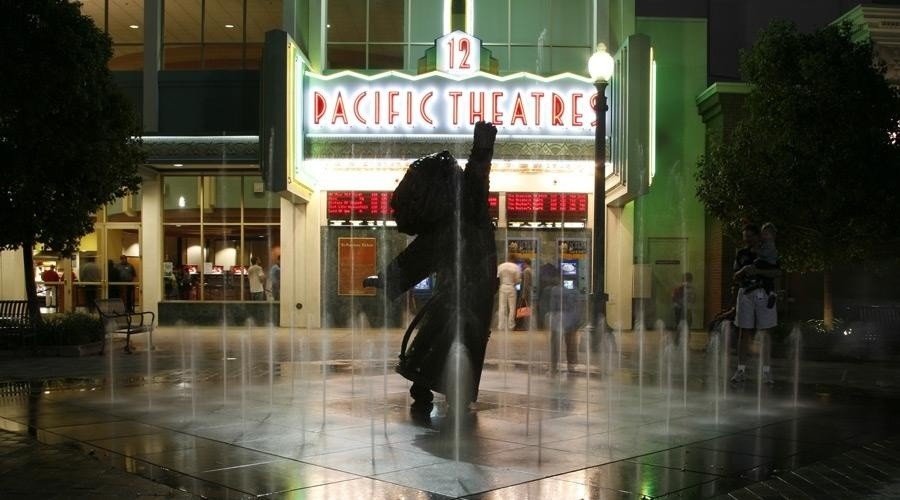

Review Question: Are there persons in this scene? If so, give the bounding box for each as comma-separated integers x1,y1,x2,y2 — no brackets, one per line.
40,254,137,315
673,272,696,349
248,257,266,301
496,254,561,332
364,121,499,423
708,284,739,352
268,256,280,300
730,224,784,384
744,223,778,309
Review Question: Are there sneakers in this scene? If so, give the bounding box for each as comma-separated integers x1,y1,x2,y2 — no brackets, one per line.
767,296,776,308
731,369,744,383
743,283,759,294
763,372,776,385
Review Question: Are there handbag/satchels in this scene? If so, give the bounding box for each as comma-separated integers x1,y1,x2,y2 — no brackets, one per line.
516,300,530,319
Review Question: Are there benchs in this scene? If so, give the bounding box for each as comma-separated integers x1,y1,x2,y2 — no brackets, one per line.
1,297,156,353
